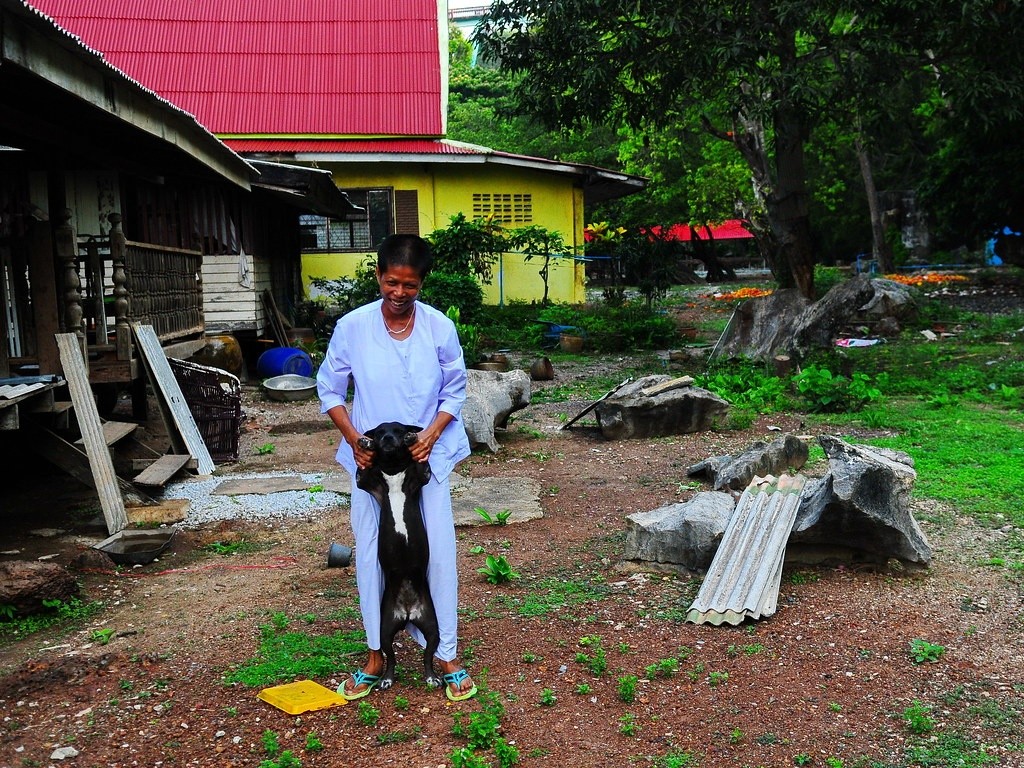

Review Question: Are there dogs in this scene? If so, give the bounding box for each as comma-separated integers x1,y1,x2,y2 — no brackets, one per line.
355,421,444,689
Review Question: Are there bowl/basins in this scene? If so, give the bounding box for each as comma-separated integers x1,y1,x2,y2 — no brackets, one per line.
262,374,317,400
92,530,176,565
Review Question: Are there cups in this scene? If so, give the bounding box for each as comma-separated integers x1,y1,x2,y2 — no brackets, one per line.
328,542,352,567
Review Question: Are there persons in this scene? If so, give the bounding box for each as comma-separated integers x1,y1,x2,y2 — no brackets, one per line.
316,234,477,701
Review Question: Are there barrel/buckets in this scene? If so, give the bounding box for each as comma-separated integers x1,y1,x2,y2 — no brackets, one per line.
257,347,314,377
530,356,554,380
491,352,506,362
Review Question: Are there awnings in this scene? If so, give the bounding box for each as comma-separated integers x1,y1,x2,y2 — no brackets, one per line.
243,157,353,217
583,219,754,246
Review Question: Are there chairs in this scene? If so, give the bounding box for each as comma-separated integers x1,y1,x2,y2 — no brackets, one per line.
166,356,241,464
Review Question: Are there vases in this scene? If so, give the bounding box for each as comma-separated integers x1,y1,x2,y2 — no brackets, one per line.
476,352,508,373
559,336,584,353
530,357,554,380
327,542,352,568
678,327,696,343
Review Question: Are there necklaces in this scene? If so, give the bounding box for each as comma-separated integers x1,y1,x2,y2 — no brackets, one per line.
382,302,415,335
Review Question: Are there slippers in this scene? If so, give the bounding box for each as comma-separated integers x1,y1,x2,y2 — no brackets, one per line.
444,669,477,702
337,668,385,700
